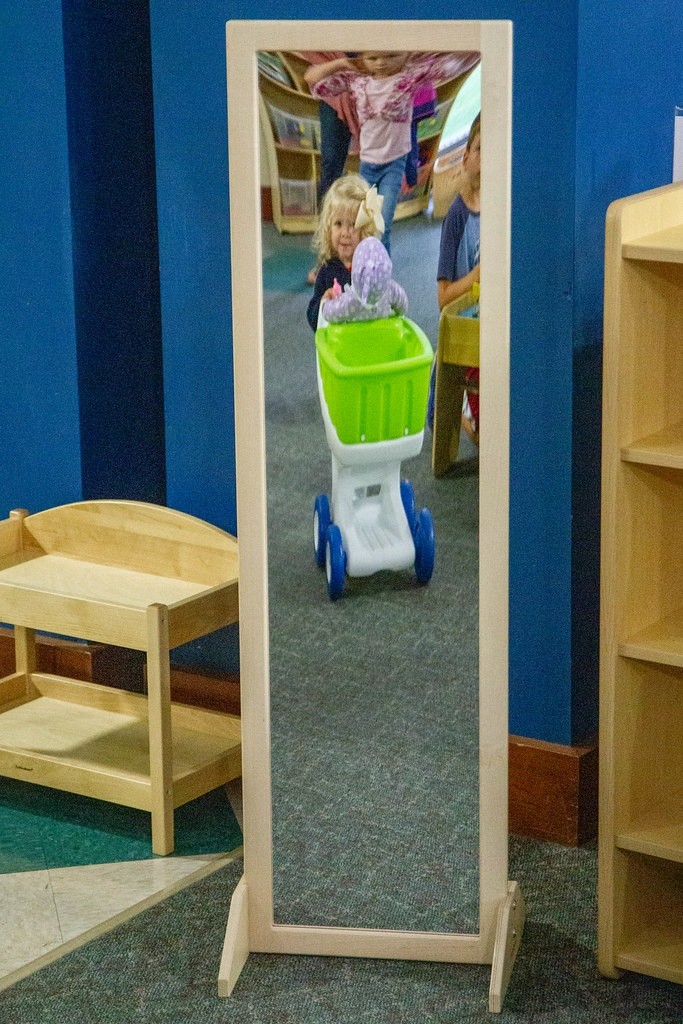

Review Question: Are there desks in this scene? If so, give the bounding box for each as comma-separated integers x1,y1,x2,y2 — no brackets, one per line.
430,279,481,479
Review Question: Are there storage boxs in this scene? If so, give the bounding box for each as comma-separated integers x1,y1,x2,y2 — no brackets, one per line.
314,316,435,444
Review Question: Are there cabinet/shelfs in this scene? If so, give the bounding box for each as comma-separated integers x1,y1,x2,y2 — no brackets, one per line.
258,57,480,236
0,499,242,856
596,174,683,991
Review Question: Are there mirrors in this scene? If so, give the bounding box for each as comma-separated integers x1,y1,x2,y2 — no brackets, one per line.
217,16,527,1015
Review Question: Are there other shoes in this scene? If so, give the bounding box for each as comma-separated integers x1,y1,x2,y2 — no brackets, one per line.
307,264,320,282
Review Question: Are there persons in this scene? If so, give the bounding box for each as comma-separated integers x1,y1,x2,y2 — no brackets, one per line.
291,52,480,283
323,237,408,324
427,110,480,444
305,172,385,332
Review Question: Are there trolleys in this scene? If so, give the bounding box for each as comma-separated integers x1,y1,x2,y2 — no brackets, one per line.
313,289,436,600
